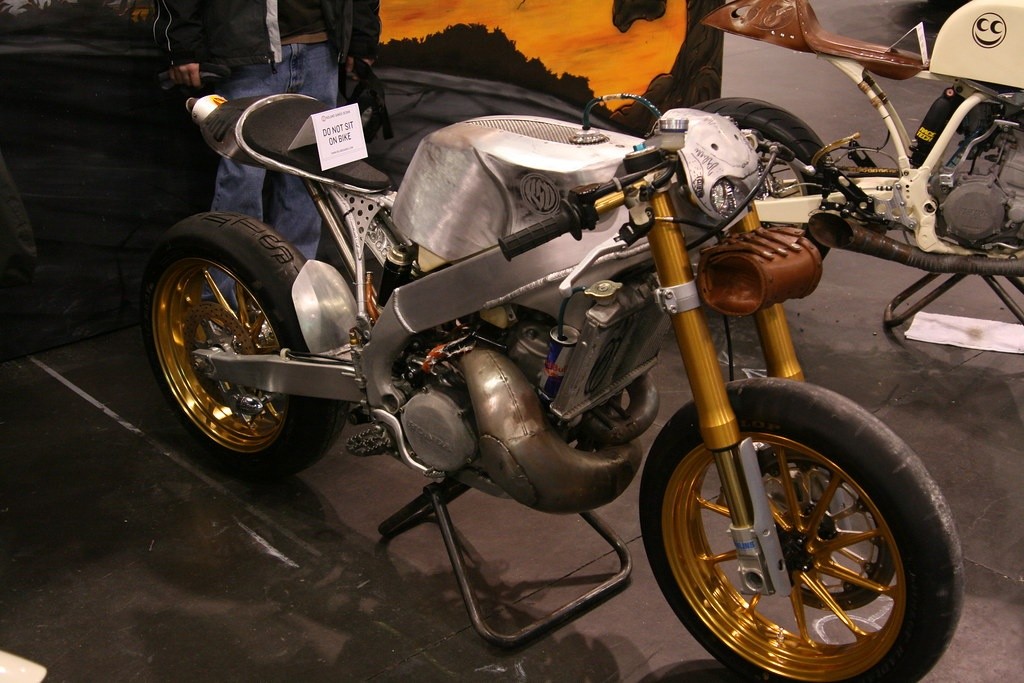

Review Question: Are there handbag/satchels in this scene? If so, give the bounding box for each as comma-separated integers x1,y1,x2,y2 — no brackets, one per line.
338,69,394,143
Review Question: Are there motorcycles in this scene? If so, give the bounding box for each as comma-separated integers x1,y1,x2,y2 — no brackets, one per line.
143,96,971,682
686,0,1024,335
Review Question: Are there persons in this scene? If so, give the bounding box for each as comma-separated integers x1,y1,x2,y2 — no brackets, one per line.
145,0,380,310
